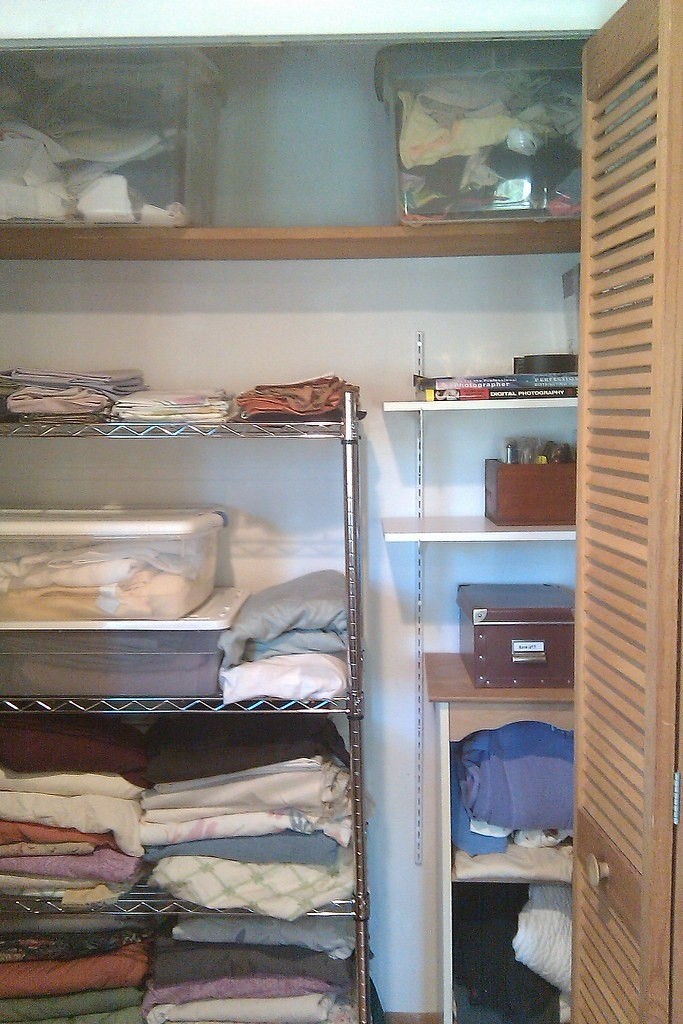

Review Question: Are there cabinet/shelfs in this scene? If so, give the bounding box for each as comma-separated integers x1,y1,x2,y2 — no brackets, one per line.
383,394,579,542
424,651,573,1024
2,386,372,1024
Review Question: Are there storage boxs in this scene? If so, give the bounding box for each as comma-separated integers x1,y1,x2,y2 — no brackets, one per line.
457,584,576,688
2,47,225,226
373,38,584,225
1,508,228,621
1,587,252,698
485,458,577,526
